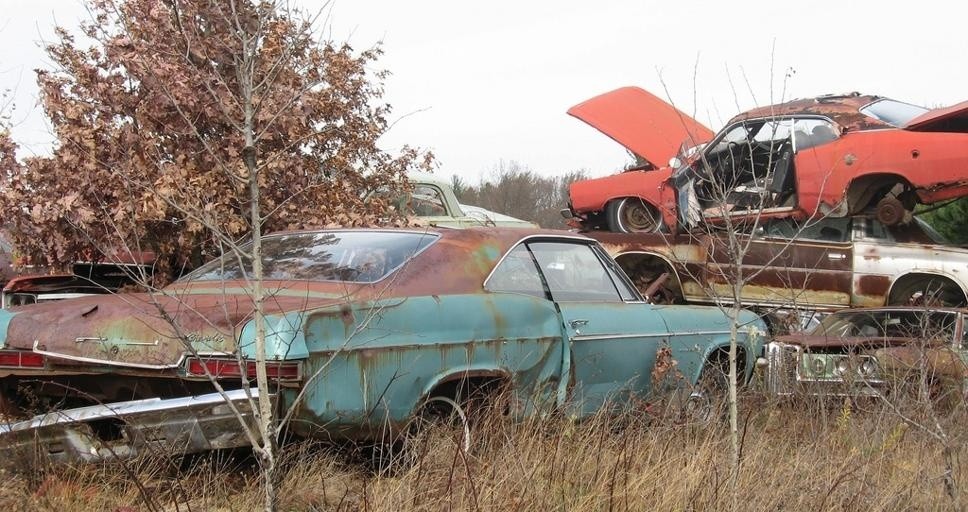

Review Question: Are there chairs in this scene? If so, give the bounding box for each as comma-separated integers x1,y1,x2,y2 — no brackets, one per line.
725,130,809,208
766,125,838,181
